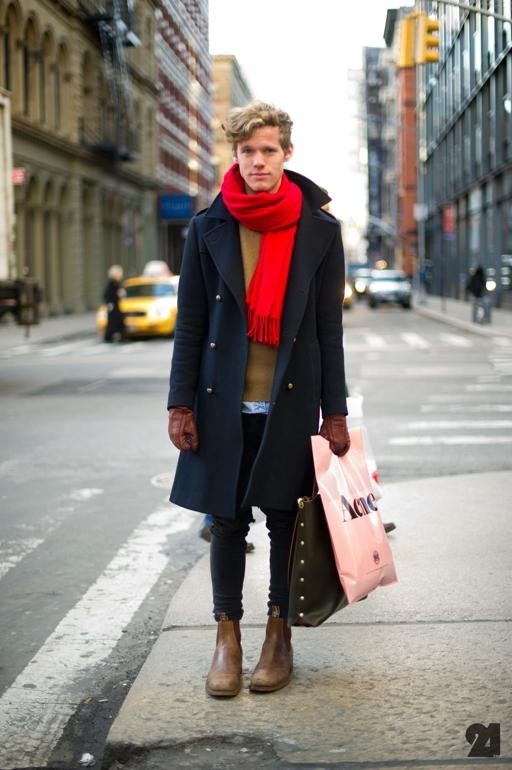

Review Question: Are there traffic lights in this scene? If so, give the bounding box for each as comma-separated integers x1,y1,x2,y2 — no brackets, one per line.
414,14,440,64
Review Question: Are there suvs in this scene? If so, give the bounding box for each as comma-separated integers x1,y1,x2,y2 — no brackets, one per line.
367,268,416,311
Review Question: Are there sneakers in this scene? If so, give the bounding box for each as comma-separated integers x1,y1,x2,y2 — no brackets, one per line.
199,526,255,553
382,520,395,533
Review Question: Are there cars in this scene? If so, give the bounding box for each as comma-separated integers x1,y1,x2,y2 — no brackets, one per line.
342,282,355,308
349,268,372,297
91,255,182,343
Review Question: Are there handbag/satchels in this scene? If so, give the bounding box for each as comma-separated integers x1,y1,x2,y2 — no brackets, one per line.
286,492,367,627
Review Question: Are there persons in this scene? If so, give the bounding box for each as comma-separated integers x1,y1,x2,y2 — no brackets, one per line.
160,101,351,700
101,262,132,343
466,263,493,326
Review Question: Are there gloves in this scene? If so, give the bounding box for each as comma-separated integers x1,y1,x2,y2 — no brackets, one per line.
169,406,199,451
318,414,350,456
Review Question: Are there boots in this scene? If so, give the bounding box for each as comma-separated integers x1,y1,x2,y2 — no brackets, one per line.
205,615,243,698
251,614,294,693
